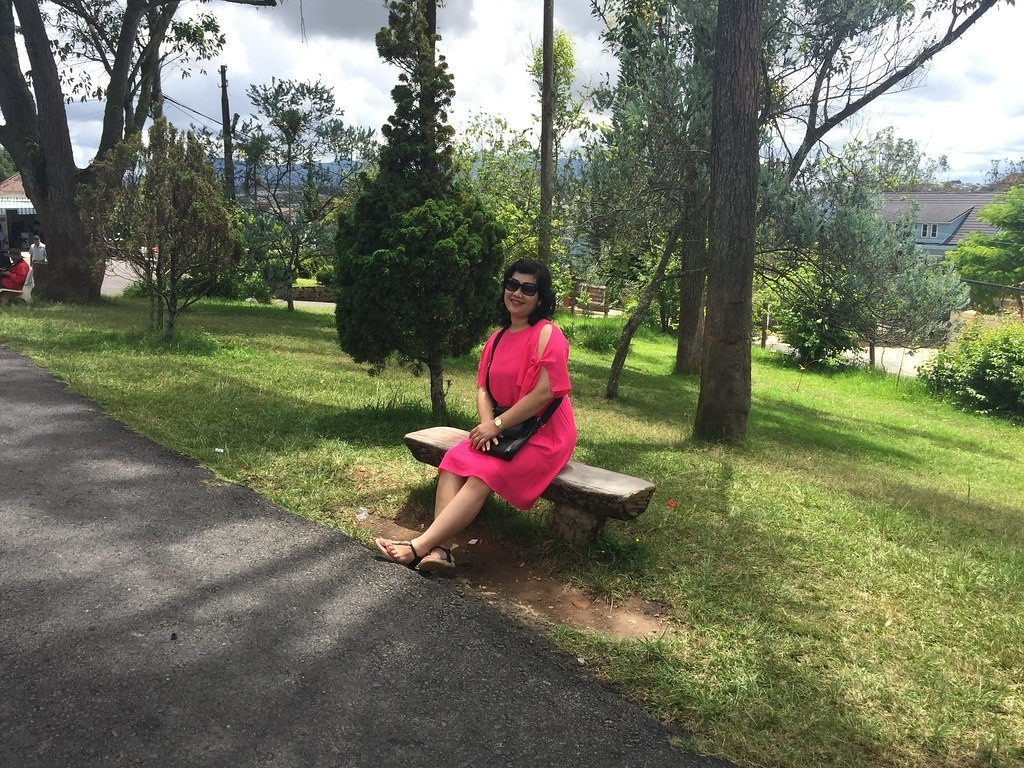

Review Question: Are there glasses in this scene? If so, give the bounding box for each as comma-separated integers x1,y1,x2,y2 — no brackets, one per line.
506,278,538,296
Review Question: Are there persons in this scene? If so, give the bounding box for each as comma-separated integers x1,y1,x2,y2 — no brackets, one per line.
0,248,29,291
29,234,47,269
376,258,576,572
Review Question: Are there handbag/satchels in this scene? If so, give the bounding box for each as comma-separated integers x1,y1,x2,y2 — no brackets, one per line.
475,407,541,462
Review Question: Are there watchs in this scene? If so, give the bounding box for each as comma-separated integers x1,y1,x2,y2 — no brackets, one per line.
494,416,505,430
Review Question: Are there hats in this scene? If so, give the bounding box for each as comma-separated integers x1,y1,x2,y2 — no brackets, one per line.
4,248,25,258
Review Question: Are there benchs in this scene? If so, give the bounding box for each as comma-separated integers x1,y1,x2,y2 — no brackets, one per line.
403,425,657,551
0,267,33,306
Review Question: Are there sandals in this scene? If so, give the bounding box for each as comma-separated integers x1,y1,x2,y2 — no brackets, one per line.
418,546,455,573
376,537,422,570
